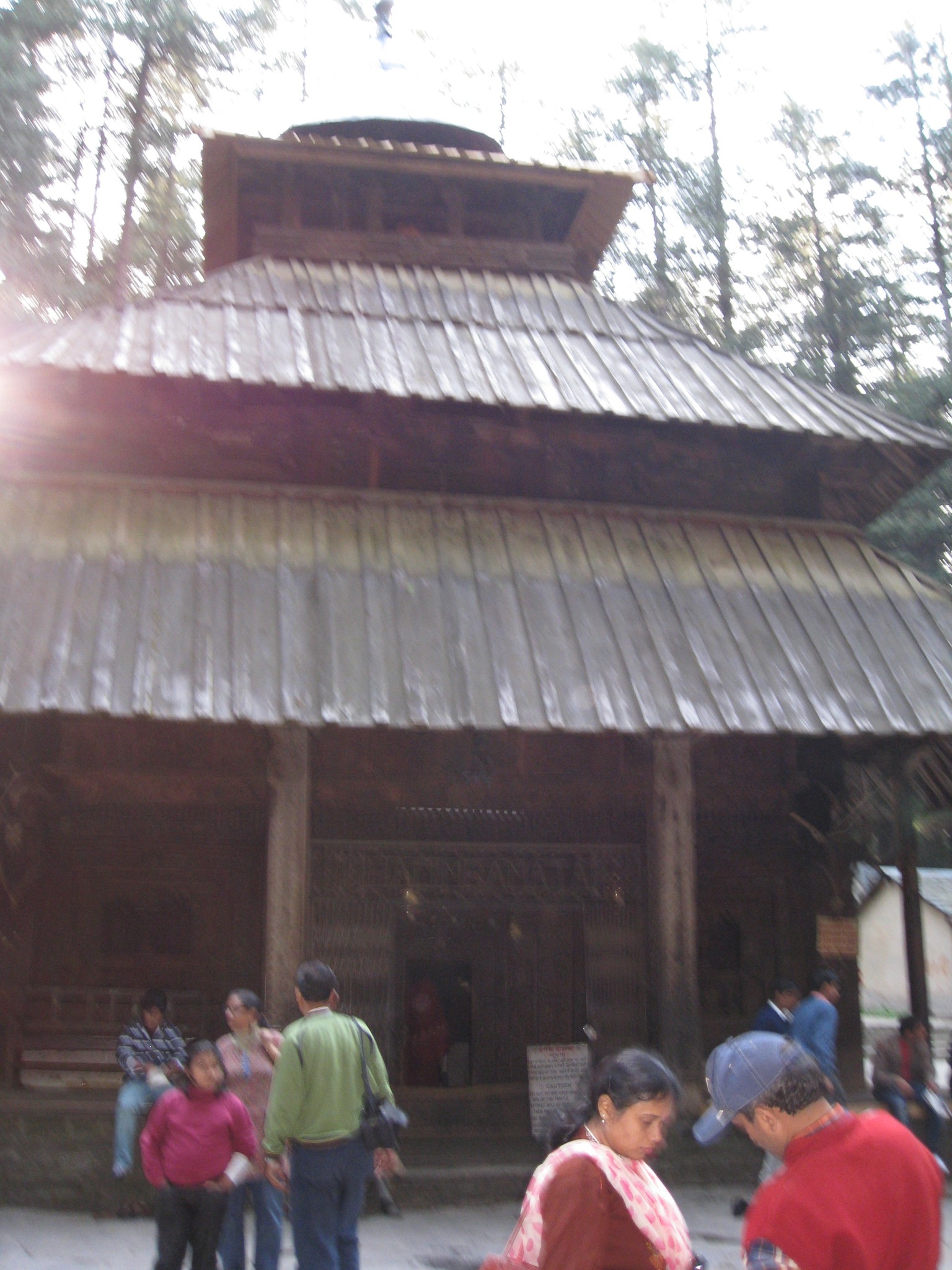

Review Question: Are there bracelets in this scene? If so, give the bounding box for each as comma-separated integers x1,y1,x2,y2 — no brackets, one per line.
264,1155,278,1161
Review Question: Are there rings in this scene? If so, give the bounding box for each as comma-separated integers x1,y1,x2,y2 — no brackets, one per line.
378,1160,382,1162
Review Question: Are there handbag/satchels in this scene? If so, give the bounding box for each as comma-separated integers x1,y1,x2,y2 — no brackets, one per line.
350,1017,409,1149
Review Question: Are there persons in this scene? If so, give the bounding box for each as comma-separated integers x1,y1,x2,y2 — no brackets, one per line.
691,1030,952,1270
479,1048,706,1270
215,987,287,1270
755,967,844,1184
261,960,397,1270
870,1014,952,1176
110,989,189,1180
142,1038,260,1270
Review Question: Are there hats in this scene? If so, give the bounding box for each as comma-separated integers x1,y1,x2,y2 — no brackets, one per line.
692,1030,802,1143
